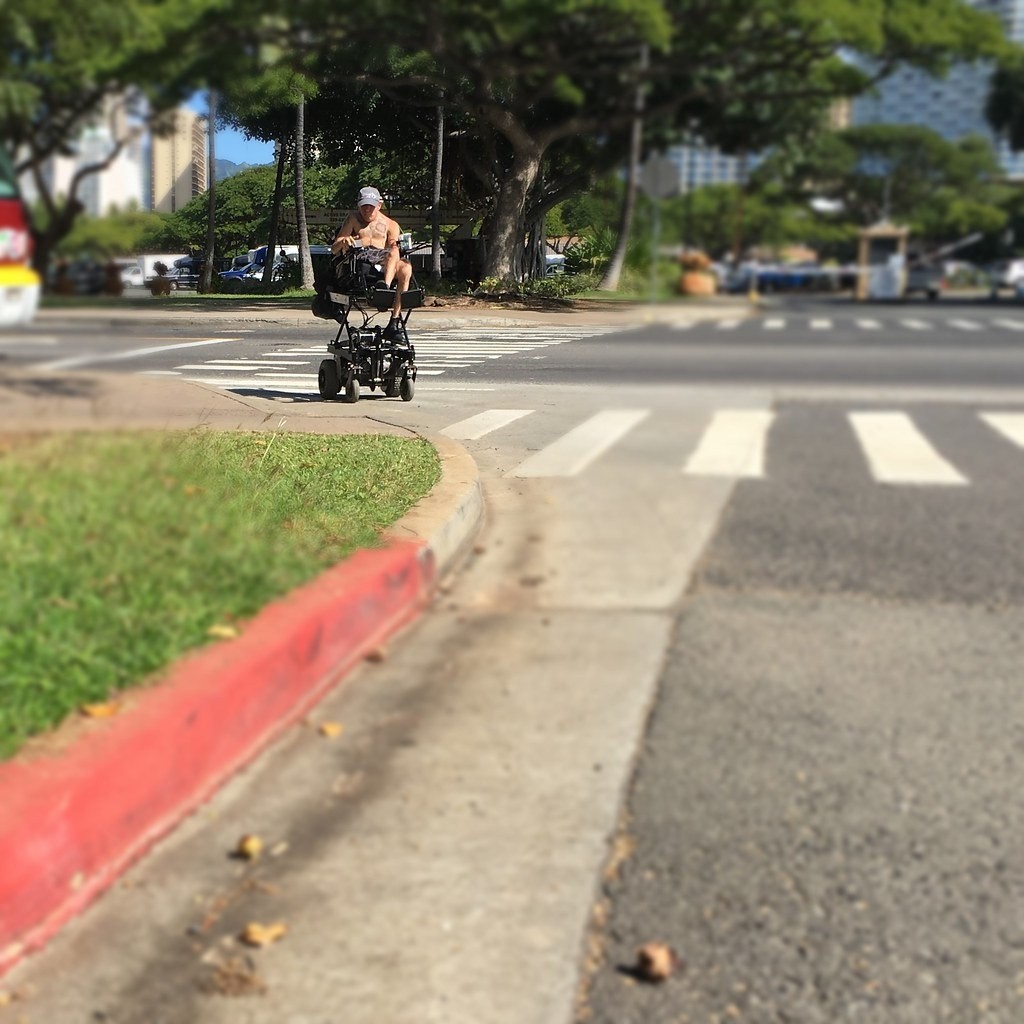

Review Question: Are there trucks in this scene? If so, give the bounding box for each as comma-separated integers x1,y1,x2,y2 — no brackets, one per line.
143,244,332,292
120,254,189,287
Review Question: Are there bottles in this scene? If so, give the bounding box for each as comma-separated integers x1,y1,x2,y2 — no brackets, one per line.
354,329,376,342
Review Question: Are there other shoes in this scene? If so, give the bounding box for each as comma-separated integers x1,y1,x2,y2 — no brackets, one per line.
376,280,390,290
383,327,406,345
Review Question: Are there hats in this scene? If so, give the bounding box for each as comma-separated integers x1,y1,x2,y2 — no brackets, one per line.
356,186,381,208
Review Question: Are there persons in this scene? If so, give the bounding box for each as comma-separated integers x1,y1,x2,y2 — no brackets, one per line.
331,187,412,346
